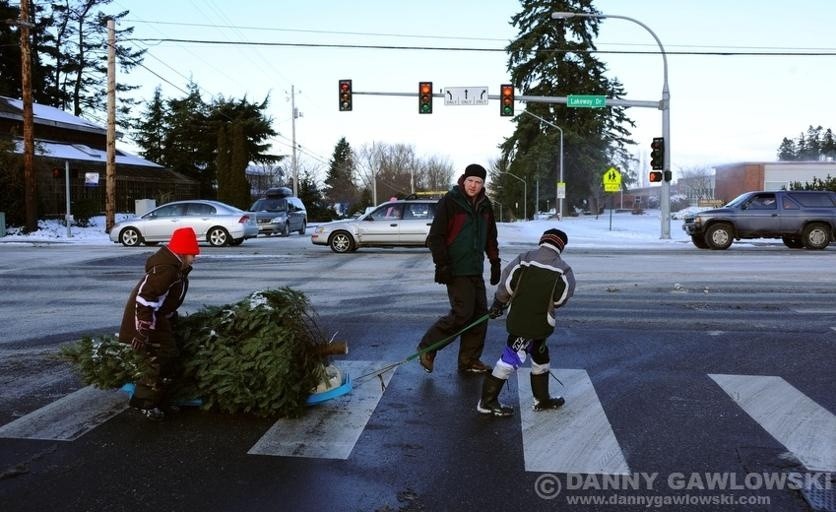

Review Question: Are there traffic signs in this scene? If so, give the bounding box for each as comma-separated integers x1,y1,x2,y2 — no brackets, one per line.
566,94,607,108
443,86,488,105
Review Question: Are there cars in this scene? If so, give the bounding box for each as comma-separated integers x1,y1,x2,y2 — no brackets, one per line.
410,203,430,218
110,199,258,246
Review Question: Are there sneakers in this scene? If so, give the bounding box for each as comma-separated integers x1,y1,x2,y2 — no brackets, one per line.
460,358,491,374
416,343,436,372
127,404,166,422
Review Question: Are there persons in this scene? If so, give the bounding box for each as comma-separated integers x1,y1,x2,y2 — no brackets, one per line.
118,228,199,422
477,229,576,418
384,206,401,219
418,165,501,374
762,197,777,209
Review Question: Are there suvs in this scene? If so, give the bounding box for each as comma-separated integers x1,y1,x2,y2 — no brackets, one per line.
683,190,836,250
251,195,308,237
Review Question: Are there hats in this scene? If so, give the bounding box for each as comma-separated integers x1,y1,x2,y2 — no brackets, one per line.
538,228,568,253
167,228,200,255
463,164,486,182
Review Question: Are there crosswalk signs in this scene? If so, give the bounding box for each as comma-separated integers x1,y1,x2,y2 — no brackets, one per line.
603,167,621,192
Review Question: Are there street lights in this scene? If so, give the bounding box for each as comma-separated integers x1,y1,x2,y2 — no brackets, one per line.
498,171,527,221
551,11,672,237
514,108,564,222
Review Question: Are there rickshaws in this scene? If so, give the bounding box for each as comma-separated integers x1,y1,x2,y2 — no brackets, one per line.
123,364,353,419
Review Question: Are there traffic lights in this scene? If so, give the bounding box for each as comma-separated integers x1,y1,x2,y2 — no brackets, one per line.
651,141,663,167
500,84,514,117
650,172,662,182
339,79,352,111
419,82,433,114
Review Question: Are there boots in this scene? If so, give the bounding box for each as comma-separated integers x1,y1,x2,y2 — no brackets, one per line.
530,371,565,411
477,369,514,416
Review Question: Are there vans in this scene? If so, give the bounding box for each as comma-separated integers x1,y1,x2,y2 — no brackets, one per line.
312,194,441,252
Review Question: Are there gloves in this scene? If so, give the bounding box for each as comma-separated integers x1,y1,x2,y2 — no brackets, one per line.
432,255,456,285
489,293,507,319
490,258,501,285
131,320,152,351
